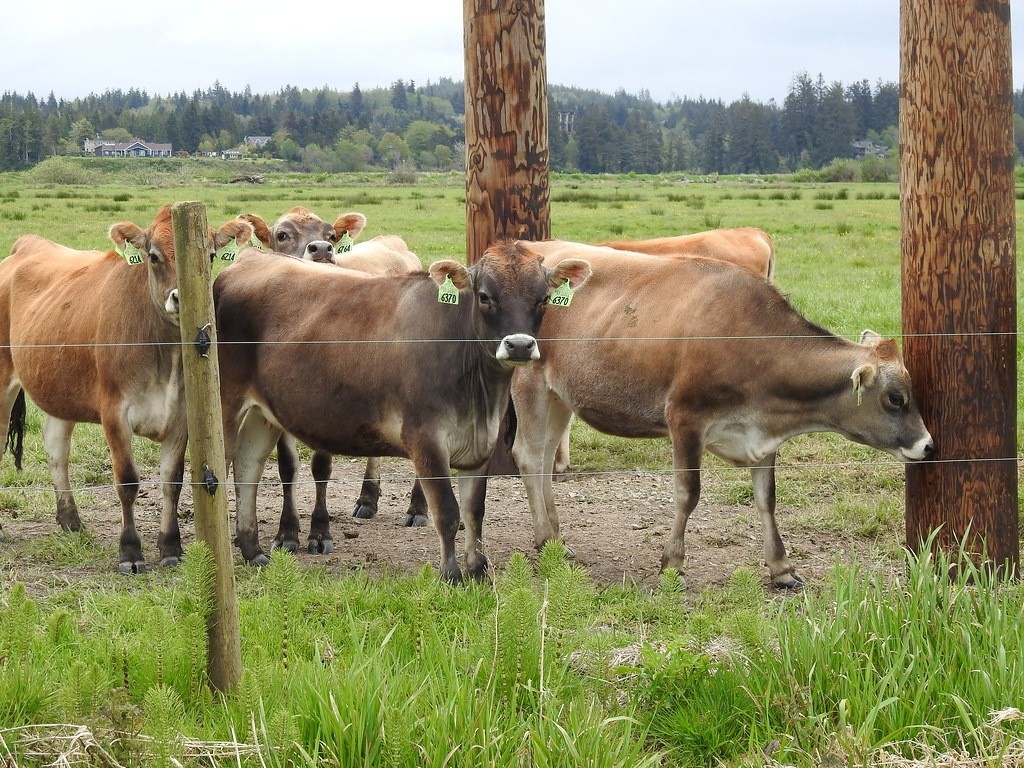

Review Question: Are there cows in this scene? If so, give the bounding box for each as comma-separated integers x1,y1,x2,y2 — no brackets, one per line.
212,241,594,586
552,227,775,474
235,207,422,557
508,236,933,590
0,202,254,574
